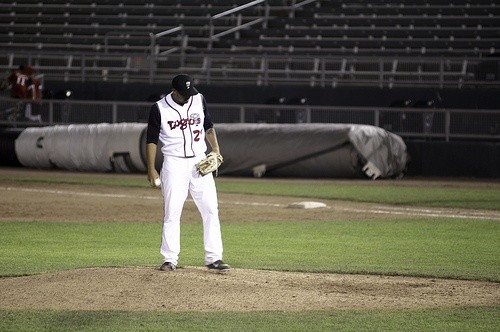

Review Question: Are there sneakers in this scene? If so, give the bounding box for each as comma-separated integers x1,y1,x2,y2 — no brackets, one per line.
160,261,176,270
207,260,230,273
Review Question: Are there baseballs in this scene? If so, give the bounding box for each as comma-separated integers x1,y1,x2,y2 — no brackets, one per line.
153,179,161,186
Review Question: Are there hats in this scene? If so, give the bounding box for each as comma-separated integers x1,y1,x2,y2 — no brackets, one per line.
172,75,198,96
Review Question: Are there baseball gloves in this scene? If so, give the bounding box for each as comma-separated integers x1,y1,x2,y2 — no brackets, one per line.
195,152,225,177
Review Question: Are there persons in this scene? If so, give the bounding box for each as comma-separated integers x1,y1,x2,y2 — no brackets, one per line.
145,74,232,270
1,63,42,101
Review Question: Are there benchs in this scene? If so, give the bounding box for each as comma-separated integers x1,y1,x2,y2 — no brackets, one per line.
0,0,500,81
